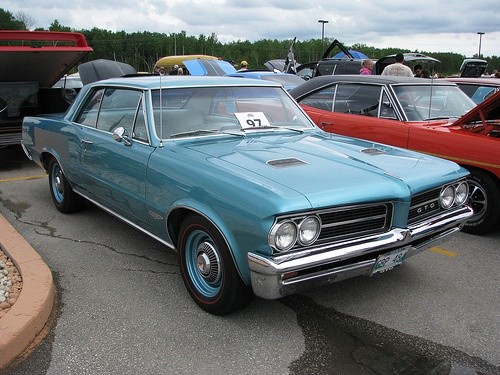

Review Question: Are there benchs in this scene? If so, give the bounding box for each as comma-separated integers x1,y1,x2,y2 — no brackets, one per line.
85,108,204,141
306,100,371,113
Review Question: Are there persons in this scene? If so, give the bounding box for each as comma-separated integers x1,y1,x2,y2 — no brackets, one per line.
169,65,180,75
414,64,430,79
177,68,183,75
238,60,249,71
359,59,373,75
159,65,169,76
381,53,414,78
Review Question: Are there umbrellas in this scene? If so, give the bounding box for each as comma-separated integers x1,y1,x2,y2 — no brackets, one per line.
332,49,369,60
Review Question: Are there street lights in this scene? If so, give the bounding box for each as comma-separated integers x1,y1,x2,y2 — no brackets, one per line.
318,19,329,46
476,31,486,59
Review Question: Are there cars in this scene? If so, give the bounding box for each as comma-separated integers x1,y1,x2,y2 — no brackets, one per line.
20,75,475,316
232,74,500,235
0,30,500,171
